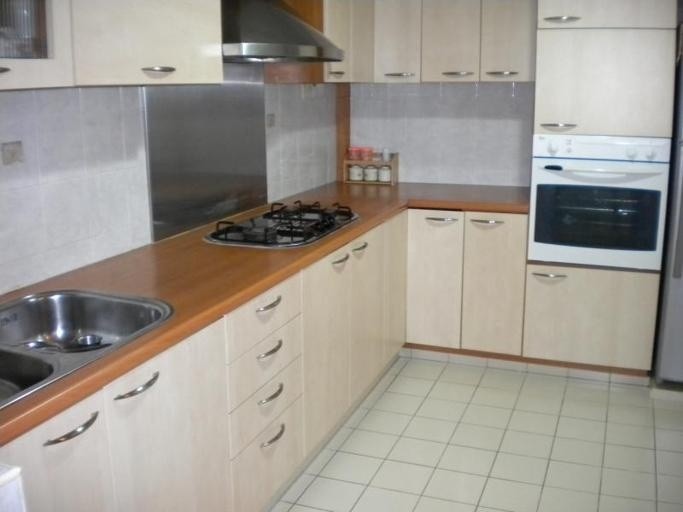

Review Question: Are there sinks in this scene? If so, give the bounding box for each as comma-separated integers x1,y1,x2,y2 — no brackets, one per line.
1,345,54,409
1,291,174,409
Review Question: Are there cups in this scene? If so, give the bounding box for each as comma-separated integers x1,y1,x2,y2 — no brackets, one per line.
348,146,391,182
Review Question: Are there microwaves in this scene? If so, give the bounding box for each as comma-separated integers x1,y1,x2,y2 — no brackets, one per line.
527,133,672,272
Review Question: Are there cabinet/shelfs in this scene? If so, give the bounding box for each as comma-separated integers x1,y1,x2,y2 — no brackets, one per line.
301,209,408,458
71,0,223,87
264,0,374,84
0,0,75,90
421,1,537,82
533,29,677,138
522,264,660,371
224,272,305,512
406,209,529,356
538,0,678,29
374,1,422,83
0,317,232,512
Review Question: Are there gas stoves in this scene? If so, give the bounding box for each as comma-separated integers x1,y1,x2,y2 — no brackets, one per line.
203,201,359,250
